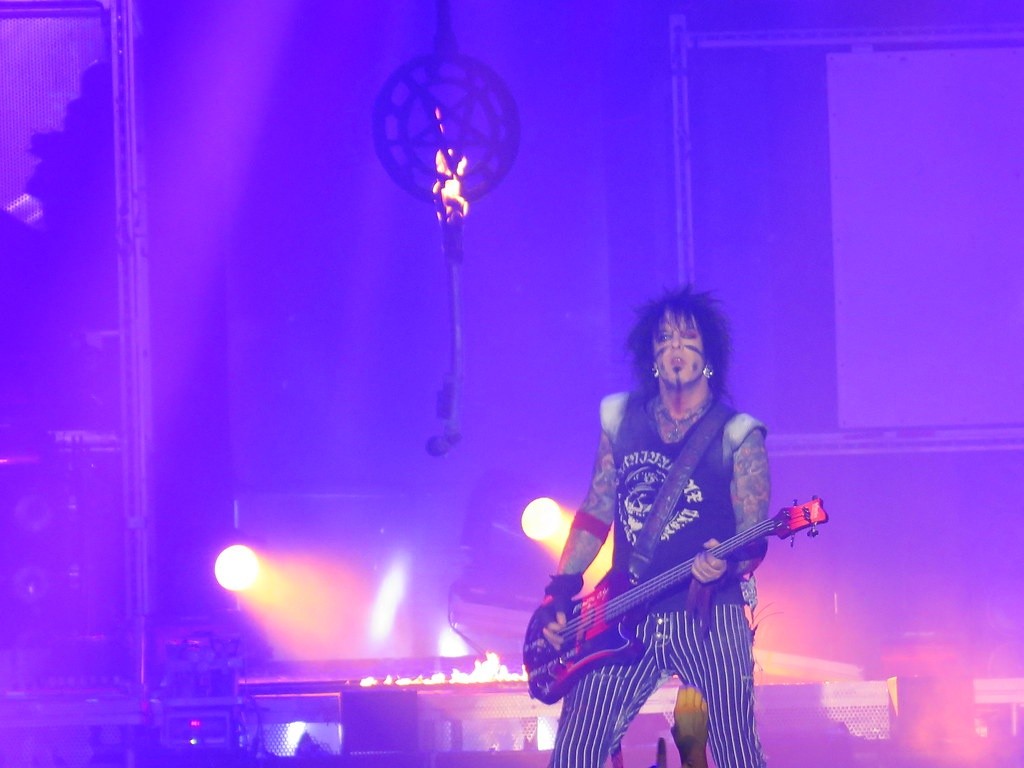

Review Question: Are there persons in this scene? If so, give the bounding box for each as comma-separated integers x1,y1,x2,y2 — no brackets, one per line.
540,272,772,768
671,684,710,768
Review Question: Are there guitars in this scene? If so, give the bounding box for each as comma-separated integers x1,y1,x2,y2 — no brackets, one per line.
522,494,828,706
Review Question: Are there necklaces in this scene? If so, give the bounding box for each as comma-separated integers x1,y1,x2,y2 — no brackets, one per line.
659,394,715,443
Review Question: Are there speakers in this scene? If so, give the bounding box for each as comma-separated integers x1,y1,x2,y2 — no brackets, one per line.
340,689,421,768
